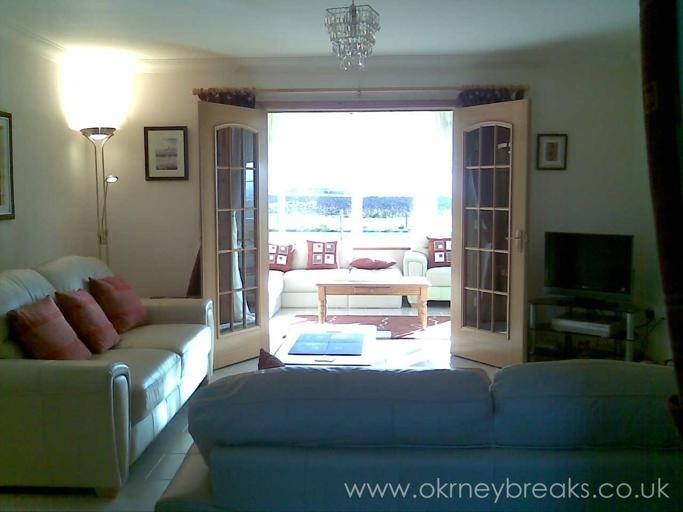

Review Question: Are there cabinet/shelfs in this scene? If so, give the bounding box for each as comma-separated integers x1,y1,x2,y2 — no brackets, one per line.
526,296,641,361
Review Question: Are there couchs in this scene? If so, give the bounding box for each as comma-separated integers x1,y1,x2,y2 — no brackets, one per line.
186,359,683,512
269,233,403,320
0,255,215,498
403,229,451,306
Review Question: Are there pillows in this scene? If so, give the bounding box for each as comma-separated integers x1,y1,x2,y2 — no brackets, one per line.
87,273,153,333
54,287,123,354
427,236,451,268
350,258,397,269
269,244,295,272
257,348,285,370
305,239,338,269
6,295,93,360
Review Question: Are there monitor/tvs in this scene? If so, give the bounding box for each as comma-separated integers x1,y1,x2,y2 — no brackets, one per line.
544,232,634,310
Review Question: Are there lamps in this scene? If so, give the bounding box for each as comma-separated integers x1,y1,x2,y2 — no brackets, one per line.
326,0,381,72
80,127,118,265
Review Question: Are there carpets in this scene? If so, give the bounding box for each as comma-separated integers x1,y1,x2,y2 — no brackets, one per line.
155,367,491,511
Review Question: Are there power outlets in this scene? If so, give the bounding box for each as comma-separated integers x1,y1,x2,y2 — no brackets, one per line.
642,304,656,323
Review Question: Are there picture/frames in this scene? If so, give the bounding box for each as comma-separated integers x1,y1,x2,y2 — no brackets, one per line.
536,133,568,170
144,126,189,181
0,110,16,220
